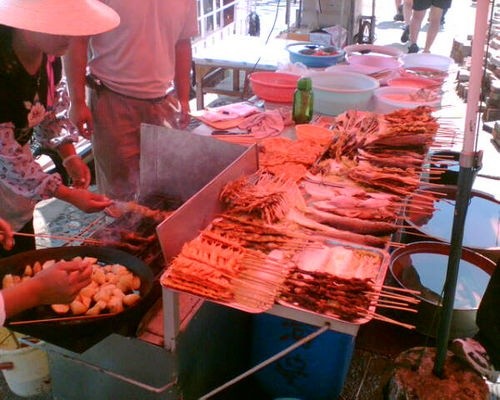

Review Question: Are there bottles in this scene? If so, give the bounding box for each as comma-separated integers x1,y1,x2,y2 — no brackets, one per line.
292,77,313,125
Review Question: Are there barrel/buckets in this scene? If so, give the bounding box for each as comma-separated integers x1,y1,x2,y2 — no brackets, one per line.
387,241,497,349
402,184,500,264
428,150,484,188
0,326,51,398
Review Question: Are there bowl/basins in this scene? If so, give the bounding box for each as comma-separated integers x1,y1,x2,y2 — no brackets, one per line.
397,52,452,73
374,87,442,114
285,42,345,67
343,45,404,63
299,71,379,117
247,72,301,103
400,67,449,85
325,64,396,85
386,77,442,90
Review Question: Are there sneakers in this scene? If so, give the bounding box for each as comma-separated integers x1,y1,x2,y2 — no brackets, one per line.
451,336,499,383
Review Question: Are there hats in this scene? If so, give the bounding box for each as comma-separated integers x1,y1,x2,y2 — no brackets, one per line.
1,0,122,37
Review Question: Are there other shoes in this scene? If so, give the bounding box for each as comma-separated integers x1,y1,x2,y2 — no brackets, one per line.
407,44,420,54
401,25,411,42
394,4,404,22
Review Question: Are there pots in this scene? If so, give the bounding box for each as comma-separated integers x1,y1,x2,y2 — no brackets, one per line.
0,244,154,339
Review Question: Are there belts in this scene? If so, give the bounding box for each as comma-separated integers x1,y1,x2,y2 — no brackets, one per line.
102,85,169,103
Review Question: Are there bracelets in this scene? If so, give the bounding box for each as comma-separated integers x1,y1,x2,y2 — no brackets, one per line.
62,154,83,167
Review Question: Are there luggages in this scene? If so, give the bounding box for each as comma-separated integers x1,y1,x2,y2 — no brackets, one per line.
247,0,260,36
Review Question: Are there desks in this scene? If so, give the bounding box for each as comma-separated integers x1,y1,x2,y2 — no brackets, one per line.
189,32,294,112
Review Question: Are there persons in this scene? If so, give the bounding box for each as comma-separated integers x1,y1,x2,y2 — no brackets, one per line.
61,0,199,204
0,0,114,260
394,0,451,53
0,217,93,327
